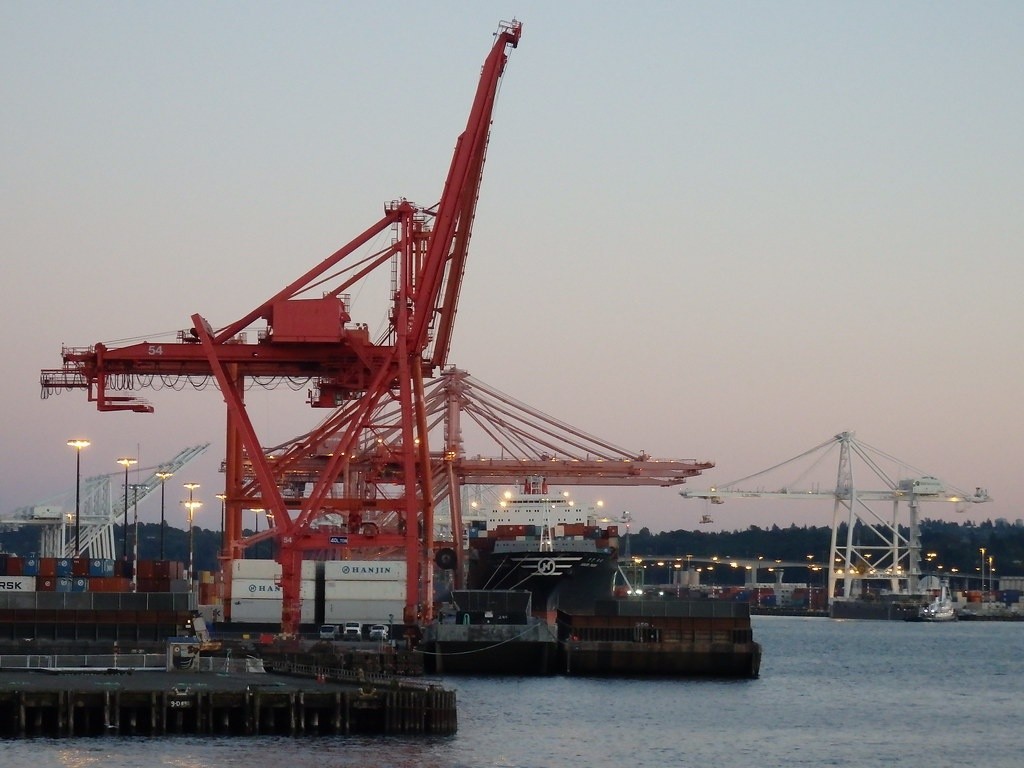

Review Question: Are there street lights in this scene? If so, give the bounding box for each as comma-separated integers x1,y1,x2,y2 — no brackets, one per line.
265,514,274,559
179,482,203,594
686,555,693,569
988,556,992,606
250,509,265,558
758,556,763,571
66,440,92,559
116,459,139,579
979,548,986,606
215,494,226,583
155,473,175,560
925,553,937,590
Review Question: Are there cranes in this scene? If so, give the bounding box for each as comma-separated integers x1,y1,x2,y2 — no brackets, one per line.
39,18,716,648
679,429,994,606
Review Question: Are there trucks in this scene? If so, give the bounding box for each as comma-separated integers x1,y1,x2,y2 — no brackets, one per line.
342,621,362,642
320,625,335,642
369,625,388,641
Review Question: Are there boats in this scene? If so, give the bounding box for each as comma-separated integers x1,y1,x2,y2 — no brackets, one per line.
922,586,955,623
470,478,619,613
